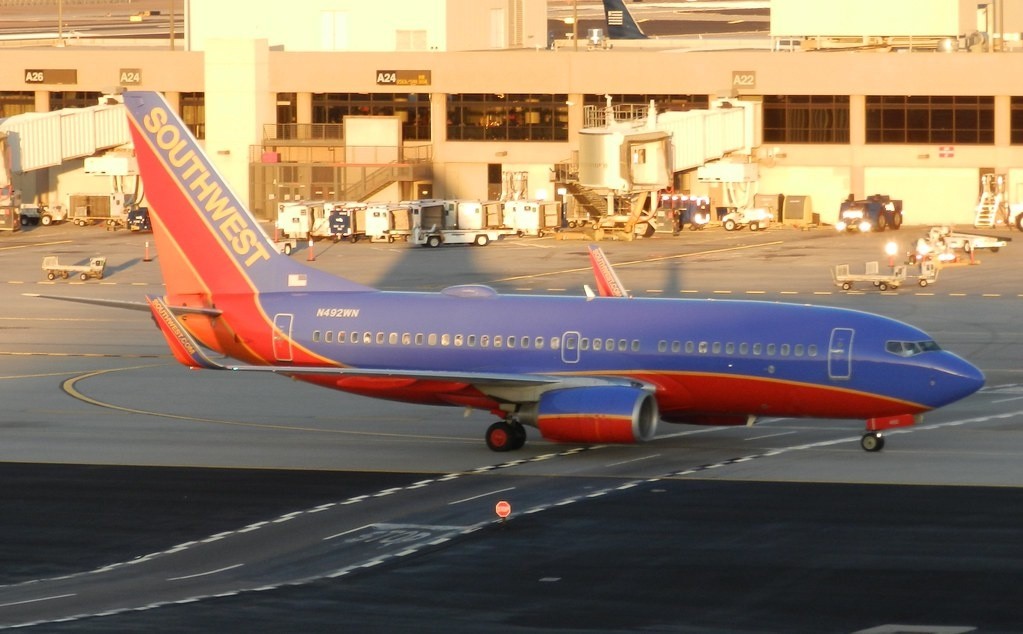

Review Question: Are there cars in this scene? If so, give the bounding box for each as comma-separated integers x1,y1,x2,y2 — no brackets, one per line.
722,209,771,234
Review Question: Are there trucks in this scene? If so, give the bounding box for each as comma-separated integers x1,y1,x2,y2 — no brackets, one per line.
125,206,150,234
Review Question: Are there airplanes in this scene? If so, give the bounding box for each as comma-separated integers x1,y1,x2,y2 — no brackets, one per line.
23,91,988,452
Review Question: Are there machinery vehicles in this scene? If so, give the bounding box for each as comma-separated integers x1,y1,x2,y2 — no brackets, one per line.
835,192,904,232
